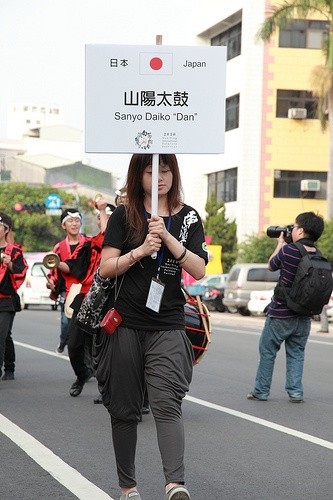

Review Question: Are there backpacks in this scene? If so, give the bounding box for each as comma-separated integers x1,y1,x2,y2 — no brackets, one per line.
276,243,333,316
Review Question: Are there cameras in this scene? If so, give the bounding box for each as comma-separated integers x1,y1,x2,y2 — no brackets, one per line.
267,225,294,244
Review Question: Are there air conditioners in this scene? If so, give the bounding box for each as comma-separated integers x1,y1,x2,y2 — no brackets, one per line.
300,180,321,191
288,107,307,118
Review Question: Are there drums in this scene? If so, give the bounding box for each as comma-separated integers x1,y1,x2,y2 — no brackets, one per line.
65,282,83,318
183,300,212,368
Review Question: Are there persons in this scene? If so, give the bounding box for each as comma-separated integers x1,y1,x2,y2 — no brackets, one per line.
246,212,324,404
47,186,151,414
0,211,29,380
92,153,209,500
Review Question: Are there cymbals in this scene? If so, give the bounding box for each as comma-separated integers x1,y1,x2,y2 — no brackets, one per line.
43,254,59,269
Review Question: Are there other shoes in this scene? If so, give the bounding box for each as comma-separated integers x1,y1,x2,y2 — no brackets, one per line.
165,483,190,500
57,343,65,353
120,488,141,500
2,373,14,380
287,395,303,403
70,378,85,397
247,391,267,401
94,395,103,404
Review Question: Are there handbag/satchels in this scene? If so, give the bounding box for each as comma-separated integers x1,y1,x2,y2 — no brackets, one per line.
72,272,115,335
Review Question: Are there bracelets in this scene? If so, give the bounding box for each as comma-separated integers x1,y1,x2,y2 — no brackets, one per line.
177,247,190,264
126,250,144,268
